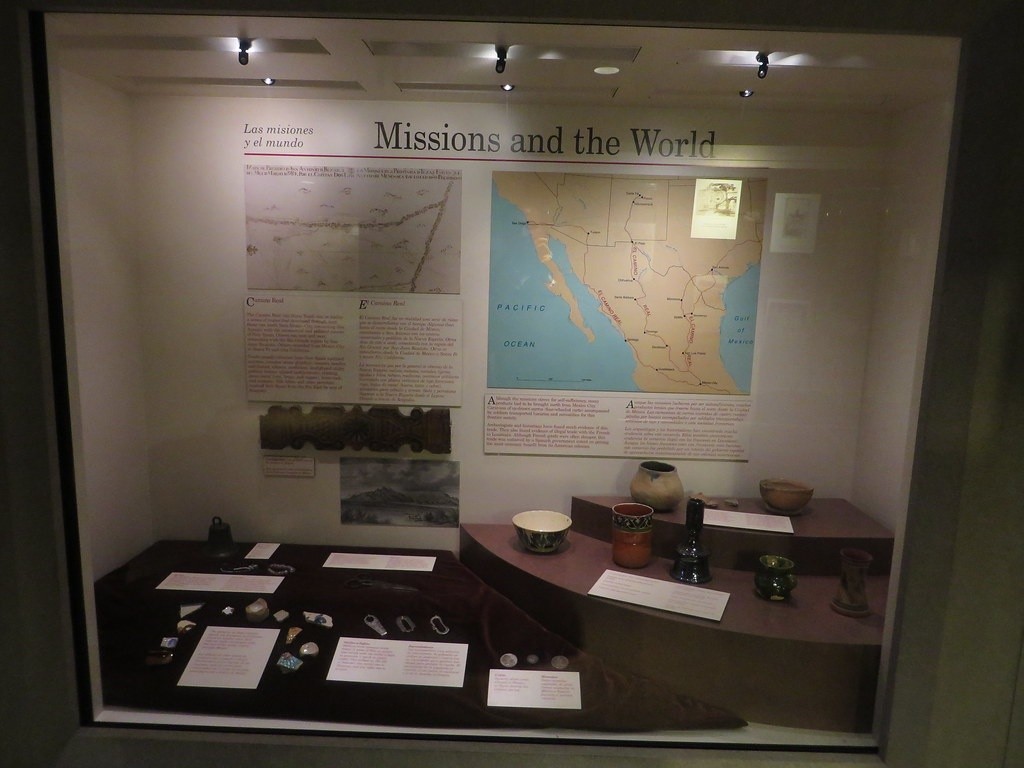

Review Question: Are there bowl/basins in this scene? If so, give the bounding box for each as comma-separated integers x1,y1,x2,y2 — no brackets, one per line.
512,510,573,553
754,555,798,600
759,479,814,511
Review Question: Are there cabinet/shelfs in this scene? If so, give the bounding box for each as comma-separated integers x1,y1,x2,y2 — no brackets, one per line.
458,498,893,736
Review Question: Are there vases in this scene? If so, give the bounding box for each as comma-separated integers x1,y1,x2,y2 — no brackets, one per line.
629,461,684,512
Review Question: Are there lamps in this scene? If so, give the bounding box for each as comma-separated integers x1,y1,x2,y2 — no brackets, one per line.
755,50,771,79
238,39,252,66
494,46,509,73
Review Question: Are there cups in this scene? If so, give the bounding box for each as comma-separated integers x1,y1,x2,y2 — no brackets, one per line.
611,503,654,568
